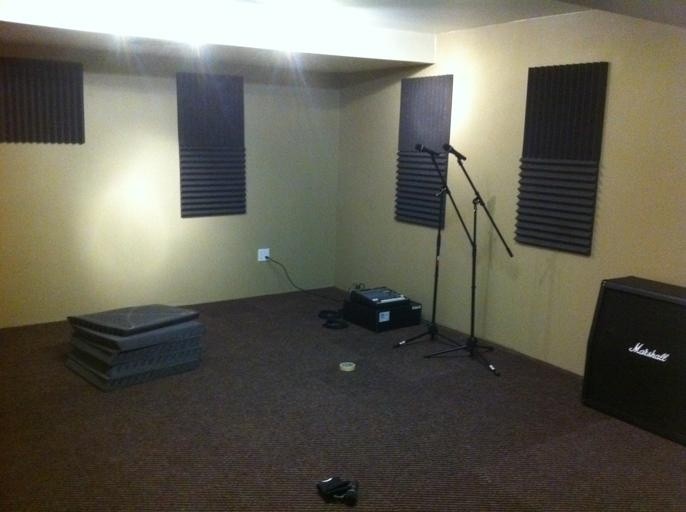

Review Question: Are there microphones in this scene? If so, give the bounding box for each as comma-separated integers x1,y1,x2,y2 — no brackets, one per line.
442,143,466,160
415,144,440,155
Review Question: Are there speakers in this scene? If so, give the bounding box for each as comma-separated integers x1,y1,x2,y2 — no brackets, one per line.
581,276,686,447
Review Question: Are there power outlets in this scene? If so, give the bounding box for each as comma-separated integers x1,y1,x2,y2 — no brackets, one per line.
258,249,270,262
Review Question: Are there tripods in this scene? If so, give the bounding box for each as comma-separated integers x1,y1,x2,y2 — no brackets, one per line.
393,156,477,348
424,160,513,376
